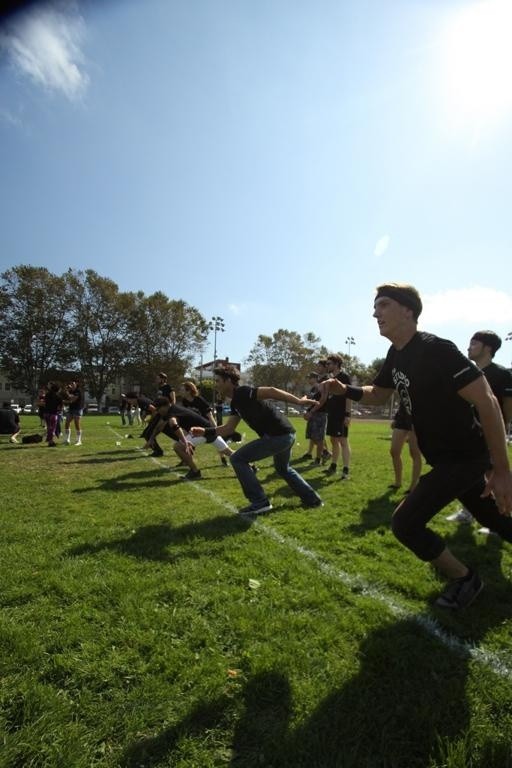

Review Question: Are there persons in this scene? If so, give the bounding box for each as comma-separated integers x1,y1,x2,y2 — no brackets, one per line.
445,328,512,537
320,282,512,616
386,397,422,494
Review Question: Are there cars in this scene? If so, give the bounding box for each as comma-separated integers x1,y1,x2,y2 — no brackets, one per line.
217,401,373,417
6,399,138,417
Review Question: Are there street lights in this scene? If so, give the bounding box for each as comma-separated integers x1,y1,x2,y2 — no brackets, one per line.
504,330,512,366
344,334,358,387
205,309,224,371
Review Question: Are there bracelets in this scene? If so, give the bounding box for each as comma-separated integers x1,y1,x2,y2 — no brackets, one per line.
344,384,363,402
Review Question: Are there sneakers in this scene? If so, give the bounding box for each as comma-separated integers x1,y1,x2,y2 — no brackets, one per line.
11,431,82,447
435,572,486,608
477,526,499,536
298,500,324,510
444,506,477,525
240,496,275,515
148,449,414,495
